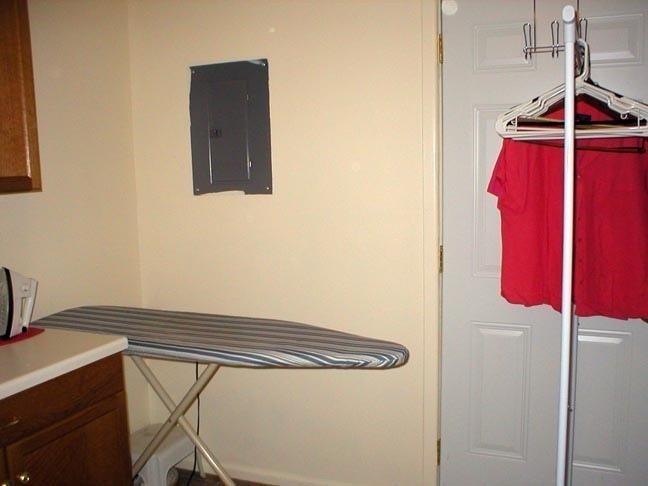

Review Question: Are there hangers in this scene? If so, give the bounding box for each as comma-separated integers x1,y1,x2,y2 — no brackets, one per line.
493,37,648,142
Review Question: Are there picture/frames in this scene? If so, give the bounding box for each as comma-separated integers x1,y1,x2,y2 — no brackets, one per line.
0,1,43,194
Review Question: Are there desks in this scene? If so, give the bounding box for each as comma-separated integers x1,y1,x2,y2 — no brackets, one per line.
27,304,408,486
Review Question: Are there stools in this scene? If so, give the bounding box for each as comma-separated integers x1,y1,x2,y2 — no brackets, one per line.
127,423,205,486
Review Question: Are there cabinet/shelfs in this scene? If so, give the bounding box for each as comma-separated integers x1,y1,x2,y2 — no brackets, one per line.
1,327,134,485
